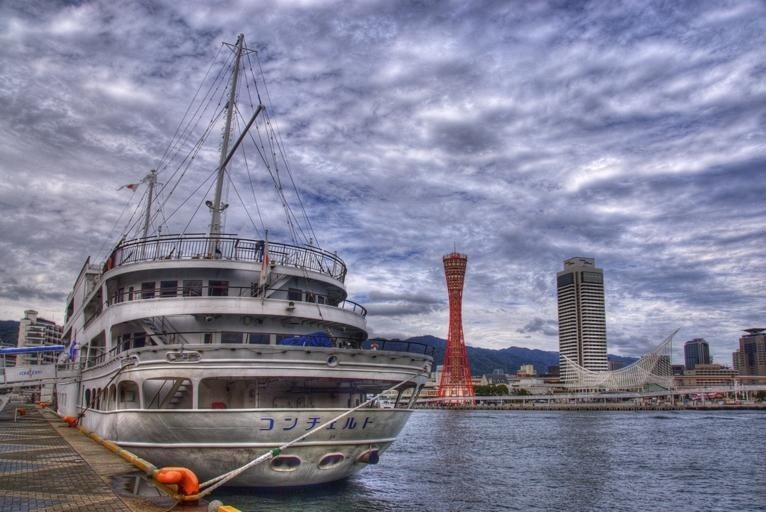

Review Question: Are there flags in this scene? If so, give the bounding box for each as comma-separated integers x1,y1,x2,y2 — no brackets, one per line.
259,239,272,288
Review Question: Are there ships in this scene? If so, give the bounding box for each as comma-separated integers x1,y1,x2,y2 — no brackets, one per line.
50,34,437,493
